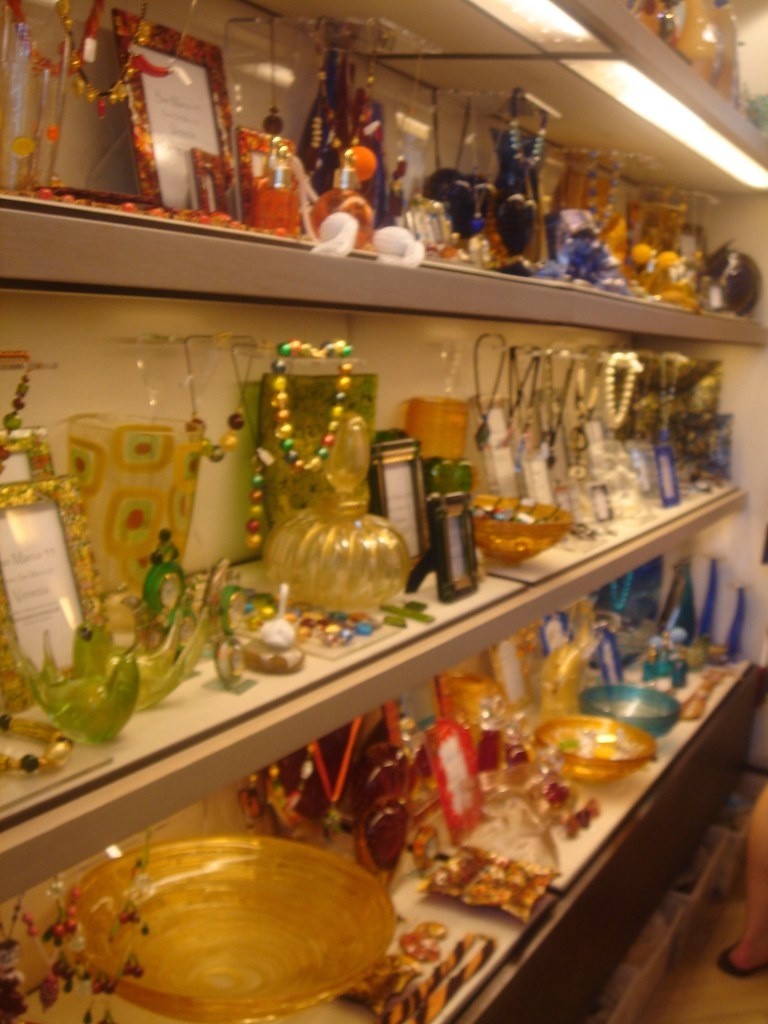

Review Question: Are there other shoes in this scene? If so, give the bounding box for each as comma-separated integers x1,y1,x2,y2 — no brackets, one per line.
717,934,767,975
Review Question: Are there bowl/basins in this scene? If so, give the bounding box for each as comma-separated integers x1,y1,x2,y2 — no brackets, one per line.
535,715,658,781
579,686,681,739
60,836,400,1021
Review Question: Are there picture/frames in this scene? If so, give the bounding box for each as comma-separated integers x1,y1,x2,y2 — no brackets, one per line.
476,394,680,522
421,619,620,838
0,473,115,714
405,491,478,604
0,426,56,486
370,438,431,569
111,7,245,226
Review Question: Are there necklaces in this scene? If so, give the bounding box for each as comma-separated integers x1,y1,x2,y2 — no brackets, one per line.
183,338,353,551
587,151,668,273
263,15,381,182
6,0,196,120
473,333,682,476
0,713,73,775
470,496,563,526
388,45,551,220
610,571,632,611
238,712,363,841
0,350,32,475
0,828,153,1024
244,588,373,647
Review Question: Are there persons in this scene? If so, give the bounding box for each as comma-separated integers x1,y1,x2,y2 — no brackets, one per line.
716,783,768,978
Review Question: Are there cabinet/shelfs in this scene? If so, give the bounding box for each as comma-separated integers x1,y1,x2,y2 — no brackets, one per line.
0,1,768,1024
